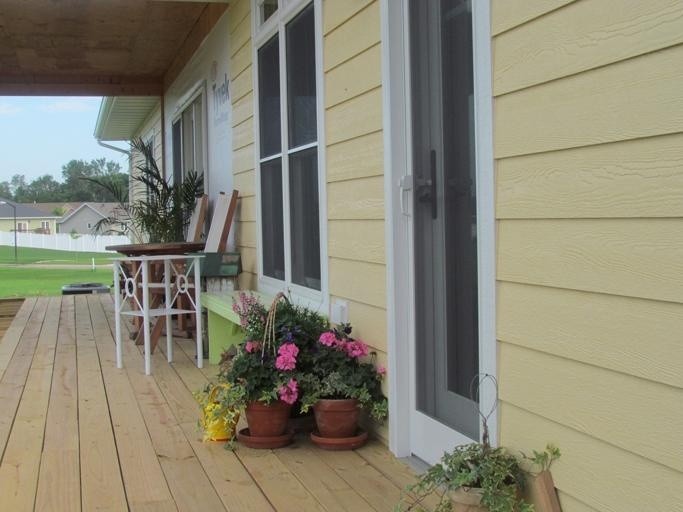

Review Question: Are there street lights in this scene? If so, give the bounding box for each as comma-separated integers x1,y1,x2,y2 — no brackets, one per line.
0,201,18,261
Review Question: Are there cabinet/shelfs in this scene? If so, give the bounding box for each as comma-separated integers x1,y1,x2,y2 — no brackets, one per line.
106,254,207,376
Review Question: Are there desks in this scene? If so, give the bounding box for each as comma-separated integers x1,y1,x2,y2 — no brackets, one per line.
105,240,206,336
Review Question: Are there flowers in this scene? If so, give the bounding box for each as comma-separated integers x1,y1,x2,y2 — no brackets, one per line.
191,289,388,451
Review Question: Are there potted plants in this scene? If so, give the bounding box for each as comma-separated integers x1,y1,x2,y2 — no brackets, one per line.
393,442,561,512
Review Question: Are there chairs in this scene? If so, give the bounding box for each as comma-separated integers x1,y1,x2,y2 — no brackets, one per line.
135,190,238,354
133,193,208,326
